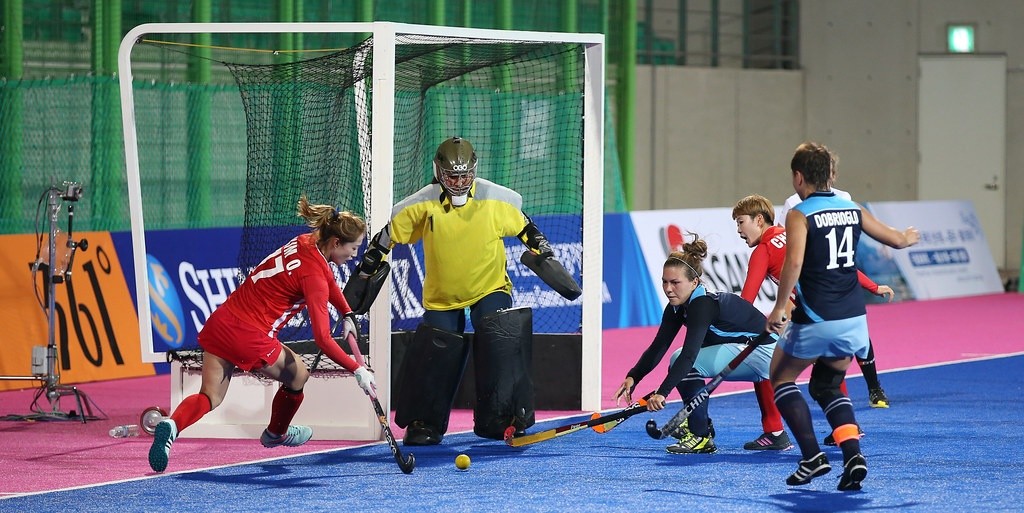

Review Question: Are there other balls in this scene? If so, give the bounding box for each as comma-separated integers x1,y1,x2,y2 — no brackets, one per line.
455,454,470,469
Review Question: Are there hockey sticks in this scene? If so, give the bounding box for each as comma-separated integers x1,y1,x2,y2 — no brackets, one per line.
646,315,787,440
504,400,666,447
368,383,416,474
591,386,661,434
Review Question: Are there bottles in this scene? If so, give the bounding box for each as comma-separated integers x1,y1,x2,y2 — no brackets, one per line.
108,423,140,439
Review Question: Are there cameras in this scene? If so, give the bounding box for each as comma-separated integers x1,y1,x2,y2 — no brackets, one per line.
64,184,84,200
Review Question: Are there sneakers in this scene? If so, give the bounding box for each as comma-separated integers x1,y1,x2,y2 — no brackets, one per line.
786,452,832,485
259,425,312,448
868,387,890,408
823,426,866,445
744,431,794,451
666,432,717,454
671,418,715,439
837,453,867,490
149,419,177,473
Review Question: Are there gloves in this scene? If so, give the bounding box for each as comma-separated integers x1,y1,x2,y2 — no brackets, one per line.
354,368,378,399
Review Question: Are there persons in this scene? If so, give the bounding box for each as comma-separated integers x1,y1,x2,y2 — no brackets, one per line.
149,196,377,472
733,194,894,452
766,141,919,491
610,233,780,455
343,138,581,446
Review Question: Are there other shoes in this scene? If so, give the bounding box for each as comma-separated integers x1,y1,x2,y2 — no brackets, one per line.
402,425,440,445
473,426,525,440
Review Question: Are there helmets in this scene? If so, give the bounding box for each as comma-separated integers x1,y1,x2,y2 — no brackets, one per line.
433,136,478,197
